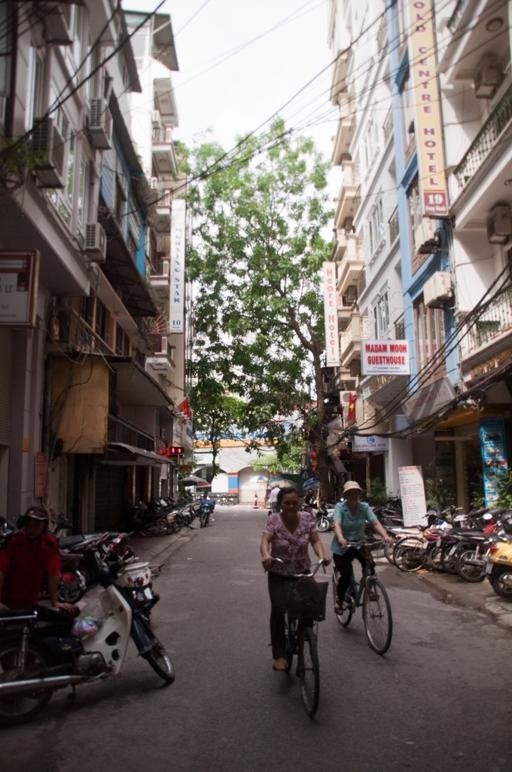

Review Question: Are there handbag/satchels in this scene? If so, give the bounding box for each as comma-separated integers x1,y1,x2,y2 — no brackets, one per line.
287,576,324,619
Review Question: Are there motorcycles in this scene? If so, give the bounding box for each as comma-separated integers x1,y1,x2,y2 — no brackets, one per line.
199,498,215,528
129,494,201,536
1,497,167,721
204,494,239,505
304,497,512,598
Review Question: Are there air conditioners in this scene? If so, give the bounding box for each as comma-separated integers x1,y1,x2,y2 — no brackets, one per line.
31,117,69,188
82,223,108,263
346,286,357,304
486,204,512,244
340,391,357,407
423,271,453,312
475,52,501,99
88,97,113,149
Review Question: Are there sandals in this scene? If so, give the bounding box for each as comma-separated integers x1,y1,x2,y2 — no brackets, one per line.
333,597,344,616
365,591,379,602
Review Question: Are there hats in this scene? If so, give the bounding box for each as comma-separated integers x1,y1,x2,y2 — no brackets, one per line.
342,480,364,494
24,506,49,520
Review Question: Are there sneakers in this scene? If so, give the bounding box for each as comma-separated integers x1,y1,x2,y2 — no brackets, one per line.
274,657,289,671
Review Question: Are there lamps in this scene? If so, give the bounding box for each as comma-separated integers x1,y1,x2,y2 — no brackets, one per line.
486,17,503,32
503,178,512,186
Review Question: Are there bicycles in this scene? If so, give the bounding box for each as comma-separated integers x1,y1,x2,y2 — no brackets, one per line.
327,535,396,655
267,554,330,713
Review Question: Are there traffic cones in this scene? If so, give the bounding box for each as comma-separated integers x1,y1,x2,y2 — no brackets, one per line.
252,490,259,510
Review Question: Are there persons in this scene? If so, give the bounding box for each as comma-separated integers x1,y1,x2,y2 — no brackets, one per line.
257,486,331,671
1,507,76,616
268,484,282,514
328,479,395,616
305,488,317,506
